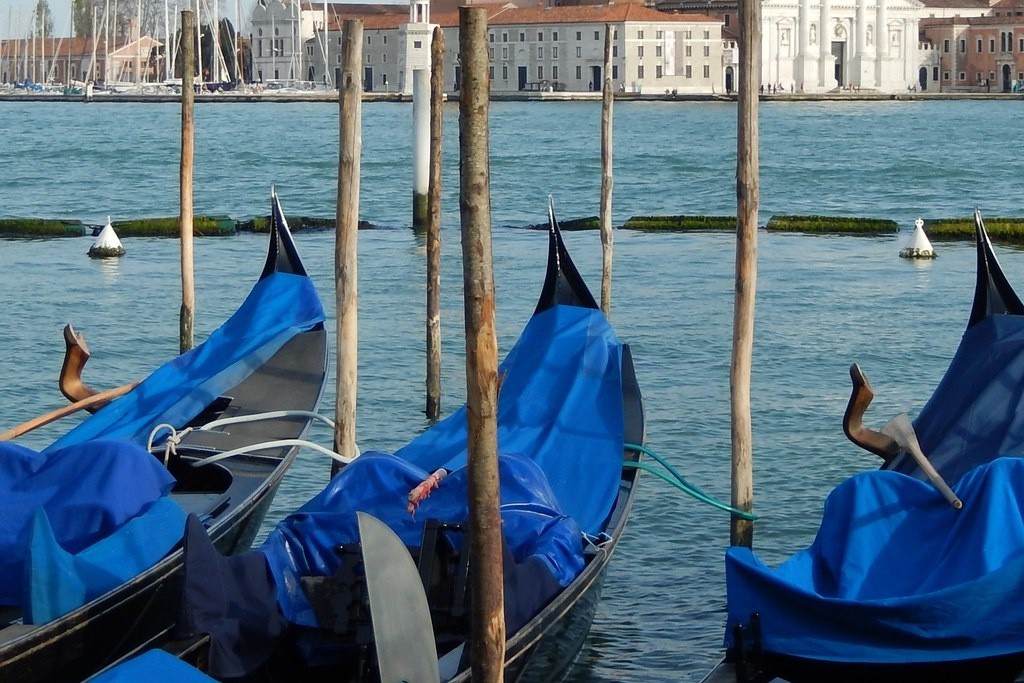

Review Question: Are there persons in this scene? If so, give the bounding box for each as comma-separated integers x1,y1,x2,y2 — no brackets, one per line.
618,81,625,91
849,82,860,92
908,84,917,93
589,81,593,91
554,80,558,90
665,89,677,97
761,82,803,94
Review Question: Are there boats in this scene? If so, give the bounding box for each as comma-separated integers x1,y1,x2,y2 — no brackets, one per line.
0,185,329,682
696,206,1024,682
89,195,644,682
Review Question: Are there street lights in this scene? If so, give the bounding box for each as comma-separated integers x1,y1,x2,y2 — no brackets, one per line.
1,0,334,90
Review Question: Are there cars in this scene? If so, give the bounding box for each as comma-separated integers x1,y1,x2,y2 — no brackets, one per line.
1013,79,1024,93
